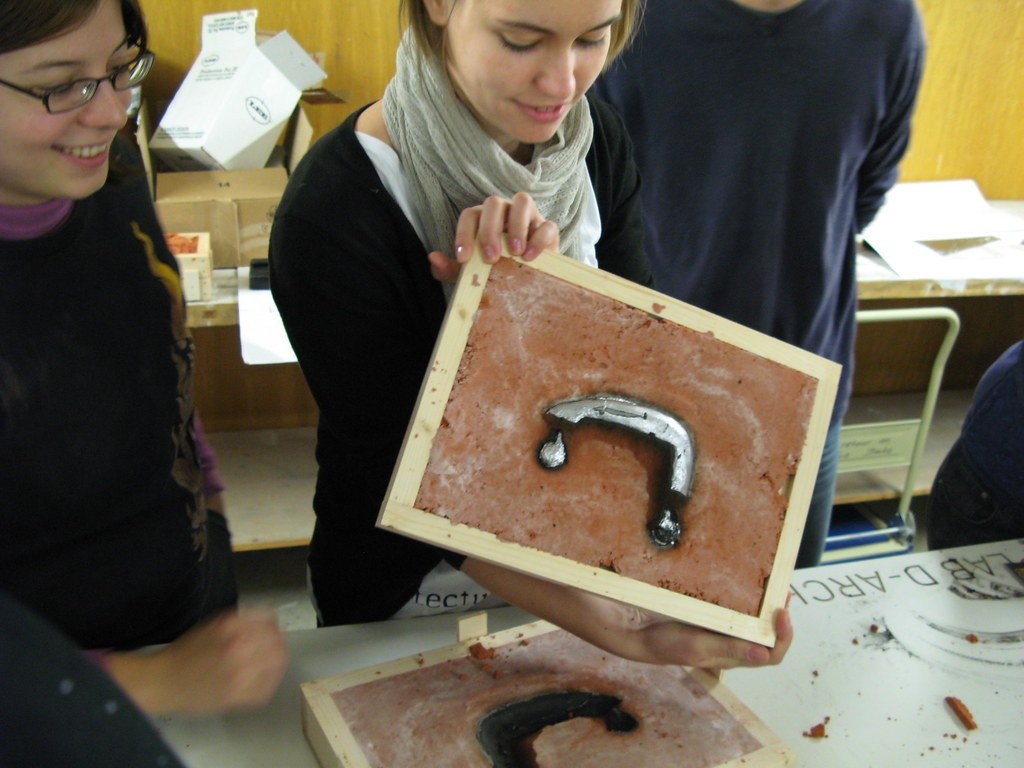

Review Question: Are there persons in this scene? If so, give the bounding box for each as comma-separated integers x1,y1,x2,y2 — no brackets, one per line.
588,0,927,568
0,0,291,768
267,0,794,669
925,339,1024,549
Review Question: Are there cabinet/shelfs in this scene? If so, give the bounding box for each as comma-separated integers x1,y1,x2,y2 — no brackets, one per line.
186,196,1024,557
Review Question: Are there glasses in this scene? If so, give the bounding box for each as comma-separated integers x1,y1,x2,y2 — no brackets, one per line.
1,42,154,113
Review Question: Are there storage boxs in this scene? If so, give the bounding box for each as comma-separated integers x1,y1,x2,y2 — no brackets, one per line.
134,9,342,302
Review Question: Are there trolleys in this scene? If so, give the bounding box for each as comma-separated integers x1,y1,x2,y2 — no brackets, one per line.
815,307,961,565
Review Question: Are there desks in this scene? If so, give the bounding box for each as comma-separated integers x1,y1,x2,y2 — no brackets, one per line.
137,536,1024,768
